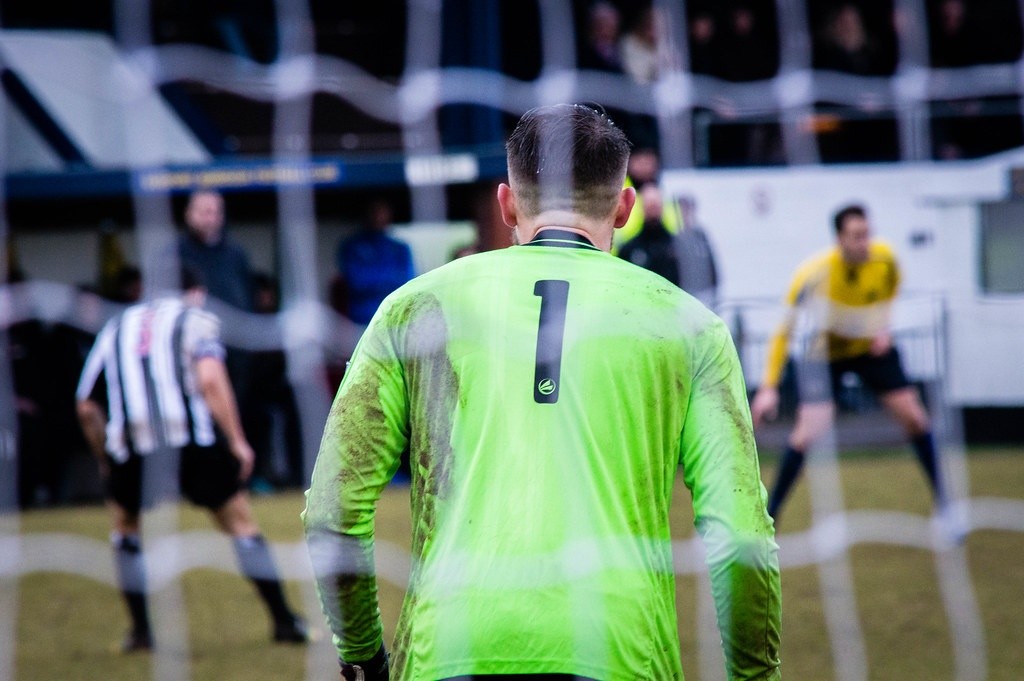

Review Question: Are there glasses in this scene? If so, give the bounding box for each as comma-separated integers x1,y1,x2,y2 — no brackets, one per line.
629,173,663,191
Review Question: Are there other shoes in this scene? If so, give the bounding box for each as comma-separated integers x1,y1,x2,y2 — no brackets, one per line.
109,631,156,656
275,622,324,645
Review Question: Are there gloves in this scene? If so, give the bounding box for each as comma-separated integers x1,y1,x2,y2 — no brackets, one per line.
338,641,391,681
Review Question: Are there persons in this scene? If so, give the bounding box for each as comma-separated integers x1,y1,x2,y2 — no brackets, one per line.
300,103,783,680
161,144,972,547
75,256,317,654
